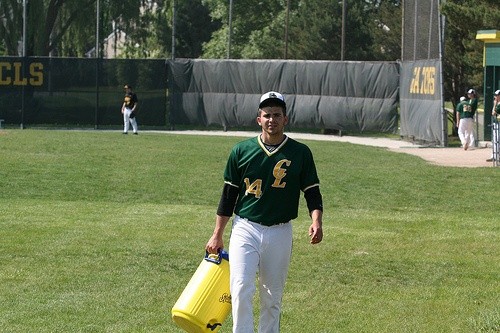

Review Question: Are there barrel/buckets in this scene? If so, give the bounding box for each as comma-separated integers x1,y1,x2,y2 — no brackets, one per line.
170,247,232,333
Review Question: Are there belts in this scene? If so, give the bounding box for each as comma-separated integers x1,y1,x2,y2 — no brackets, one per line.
240,216,289,226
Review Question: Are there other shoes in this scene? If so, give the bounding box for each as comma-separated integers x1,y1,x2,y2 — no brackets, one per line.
486,158,495,161
464,142,468,150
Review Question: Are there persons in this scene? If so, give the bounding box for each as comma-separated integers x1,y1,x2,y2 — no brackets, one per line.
205,91,323,333
456,89,500,163
121,85,139,134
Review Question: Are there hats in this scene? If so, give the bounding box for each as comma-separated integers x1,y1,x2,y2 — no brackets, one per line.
460,97,466,102
494,90,500,94
258,91,286,109
467,89,475,94
124,85,131,89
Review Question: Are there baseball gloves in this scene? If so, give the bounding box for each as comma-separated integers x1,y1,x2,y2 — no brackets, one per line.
129,111,135,119
